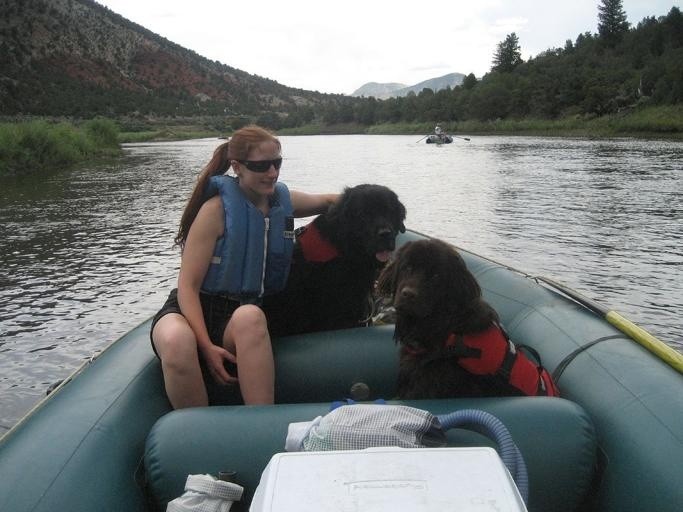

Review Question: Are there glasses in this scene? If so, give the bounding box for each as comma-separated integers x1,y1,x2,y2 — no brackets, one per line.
235,157,282,171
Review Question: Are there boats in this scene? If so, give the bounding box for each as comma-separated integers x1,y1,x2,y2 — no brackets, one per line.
426,134,452,144
0,228,683,511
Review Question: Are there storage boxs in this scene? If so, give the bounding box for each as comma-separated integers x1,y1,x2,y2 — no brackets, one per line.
247,445,531,510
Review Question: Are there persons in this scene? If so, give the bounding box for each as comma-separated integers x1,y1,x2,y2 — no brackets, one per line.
151,125,337,409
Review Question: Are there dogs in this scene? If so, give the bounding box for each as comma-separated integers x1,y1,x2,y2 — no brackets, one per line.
261,183,408,339
371,236,563,398
